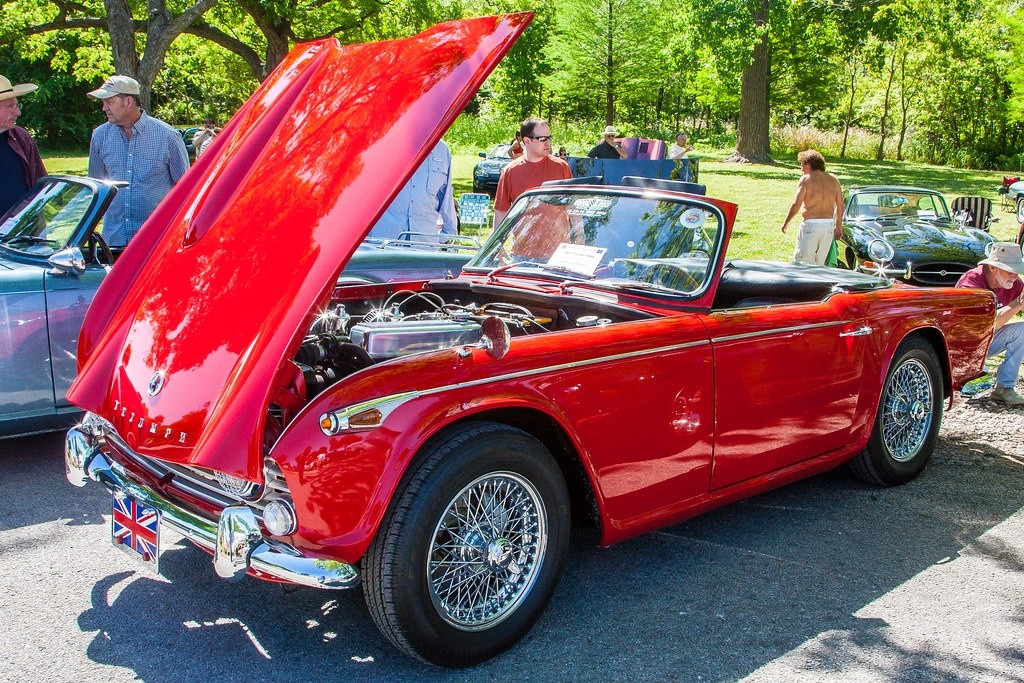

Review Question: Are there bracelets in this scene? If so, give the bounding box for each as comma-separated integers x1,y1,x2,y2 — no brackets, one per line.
1017,296,1020,305
614,144,619,149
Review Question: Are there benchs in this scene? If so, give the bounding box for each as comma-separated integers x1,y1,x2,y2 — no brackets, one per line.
857,204,906,215
625,260,834,303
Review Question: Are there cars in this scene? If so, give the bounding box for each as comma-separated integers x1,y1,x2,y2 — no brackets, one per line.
472,144,526,195
0,174,518,434
66,12,999,674
1007,181,1024,225
824,184,1001,288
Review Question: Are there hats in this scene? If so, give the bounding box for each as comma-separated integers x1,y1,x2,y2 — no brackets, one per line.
600,126,620,137
0,75,39,102
87,75,140,99
977,242,1024,276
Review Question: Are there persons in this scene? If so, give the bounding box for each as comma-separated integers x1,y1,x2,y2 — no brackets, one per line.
364,139,457,250
86,76,190,246
954,242,1024,404
192,118,216,160
667,132,692,159
586,126,627,159
0,74,63,244
781,150,845,265
559,146,568,161
492,118,584,268
508,131,523,161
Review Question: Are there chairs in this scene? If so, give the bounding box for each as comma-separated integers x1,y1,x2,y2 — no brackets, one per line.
951,195,1001,235
998,176,1021,214
459,193,491,241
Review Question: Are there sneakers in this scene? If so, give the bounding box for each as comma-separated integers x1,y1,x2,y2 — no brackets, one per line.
990,383,1024,404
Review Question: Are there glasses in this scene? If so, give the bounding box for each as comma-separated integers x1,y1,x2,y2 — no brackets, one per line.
0,103,23,111
524,133,553,142
